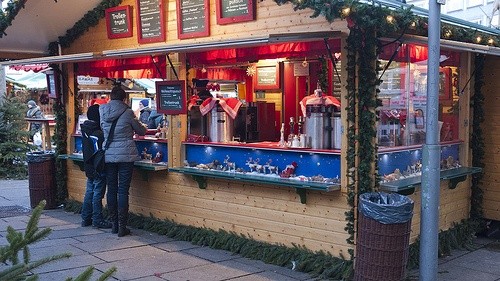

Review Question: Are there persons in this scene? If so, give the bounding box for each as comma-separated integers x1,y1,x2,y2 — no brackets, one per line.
99,86,146,237
26,101,44,141
80,105,112,229
135,95,164,159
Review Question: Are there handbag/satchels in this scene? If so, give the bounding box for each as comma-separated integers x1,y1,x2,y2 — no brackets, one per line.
86,148,105,173
33,131,42,145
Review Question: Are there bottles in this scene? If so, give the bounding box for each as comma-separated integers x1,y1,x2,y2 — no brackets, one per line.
292,133,309,147
316,80,322,89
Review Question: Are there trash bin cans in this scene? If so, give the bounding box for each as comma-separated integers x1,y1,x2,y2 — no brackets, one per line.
26,151,60,210
355,191,414,281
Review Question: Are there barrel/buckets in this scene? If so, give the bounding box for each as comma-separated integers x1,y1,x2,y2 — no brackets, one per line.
207,104,236,143
301,104,344,150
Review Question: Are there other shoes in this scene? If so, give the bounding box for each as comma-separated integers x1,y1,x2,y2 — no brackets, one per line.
92,221,112,229
82,218,93,227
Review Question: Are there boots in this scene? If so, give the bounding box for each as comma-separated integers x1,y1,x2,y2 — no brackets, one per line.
109,209,118,234
118,207,131,237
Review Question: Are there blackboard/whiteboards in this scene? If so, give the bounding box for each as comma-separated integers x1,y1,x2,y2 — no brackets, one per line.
155,79,188,114
104,0,256,45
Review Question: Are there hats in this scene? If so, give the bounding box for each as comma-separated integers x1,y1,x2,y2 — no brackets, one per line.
141,99,149,107
87,104,100,121
